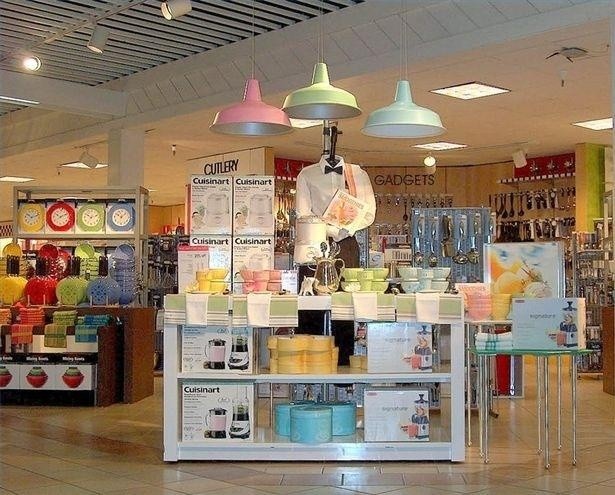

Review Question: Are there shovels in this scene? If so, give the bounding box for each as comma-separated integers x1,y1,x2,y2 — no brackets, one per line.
441,214,455,257
494,194,526,219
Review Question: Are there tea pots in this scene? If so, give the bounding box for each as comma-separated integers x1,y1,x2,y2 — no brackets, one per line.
307,255,345,293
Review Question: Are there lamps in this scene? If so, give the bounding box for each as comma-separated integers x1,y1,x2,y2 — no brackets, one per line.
208,0,448,139
423,151,436,175
87,0,193,54
79,148,99,169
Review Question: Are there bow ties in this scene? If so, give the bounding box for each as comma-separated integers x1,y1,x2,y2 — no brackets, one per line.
324,165,342,174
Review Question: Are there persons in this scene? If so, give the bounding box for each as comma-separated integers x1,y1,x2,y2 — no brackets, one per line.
295,154,368,362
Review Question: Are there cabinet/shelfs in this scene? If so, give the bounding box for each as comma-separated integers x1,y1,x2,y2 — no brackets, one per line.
163,295,465,464
13,190,140,239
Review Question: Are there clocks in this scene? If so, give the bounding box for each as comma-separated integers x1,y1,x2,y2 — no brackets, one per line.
17,202,135,231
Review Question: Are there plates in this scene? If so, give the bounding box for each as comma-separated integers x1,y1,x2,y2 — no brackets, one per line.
17,202,135,232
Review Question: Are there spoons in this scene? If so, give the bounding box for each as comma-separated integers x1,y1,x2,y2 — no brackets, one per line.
403,198,408,221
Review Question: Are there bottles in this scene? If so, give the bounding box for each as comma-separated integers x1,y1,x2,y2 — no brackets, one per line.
204,338,227,370
204,408,227,438
229,334,250,370
2,255,121,306
229,404,249,438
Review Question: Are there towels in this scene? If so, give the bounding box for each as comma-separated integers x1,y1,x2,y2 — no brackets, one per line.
0,307,114,348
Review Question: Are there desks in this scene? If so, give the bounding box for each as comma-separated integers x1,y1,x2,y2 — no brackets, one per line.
467,347,594,471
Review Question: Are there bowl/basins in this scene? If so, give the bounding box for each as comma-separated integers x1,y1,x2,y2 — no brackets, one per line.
1,240,135,261
196,268,282,294
0,375,86,388
110,256,138,305
338,264,451,295
